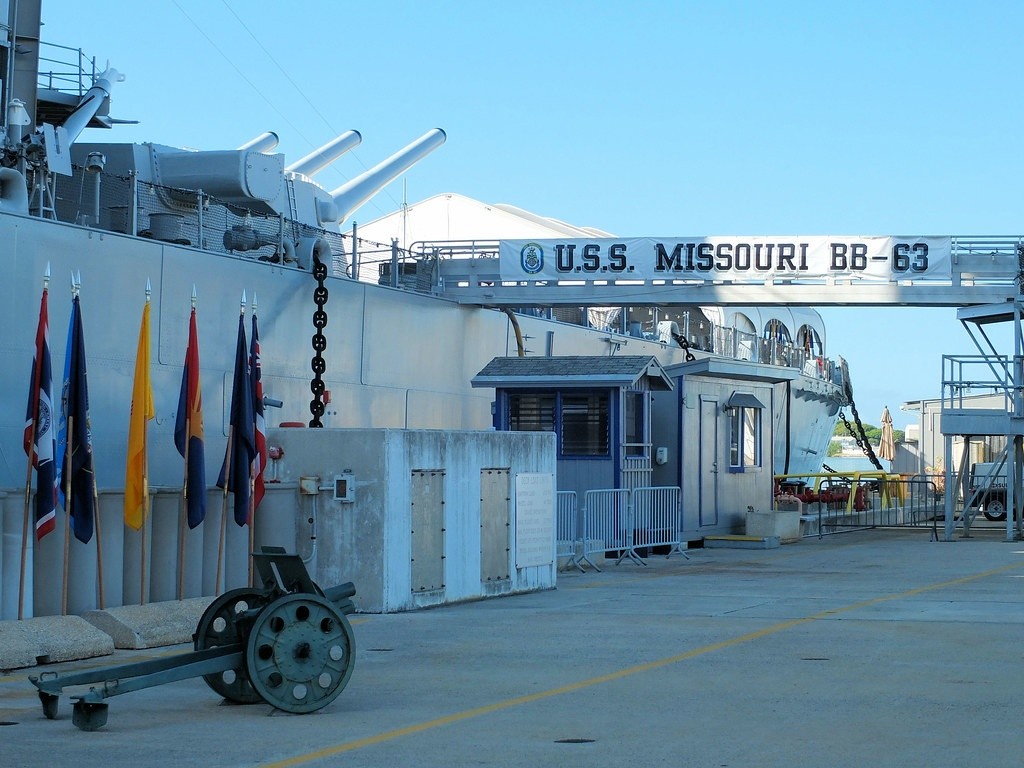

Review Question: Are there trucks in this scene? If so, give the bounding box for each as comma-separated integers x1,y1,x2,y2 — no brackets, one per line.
968,462,1024,521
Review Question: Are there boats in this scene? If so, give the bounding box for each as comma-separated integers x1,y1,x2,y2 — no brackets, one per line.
0,0,853,493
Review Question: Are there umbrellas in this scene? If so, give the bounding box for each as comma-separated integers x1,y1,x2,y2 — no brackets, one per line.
877,405,895,473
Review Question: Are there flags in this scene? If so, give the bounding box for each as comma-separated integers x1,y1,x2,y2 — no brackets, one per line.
246,324,267,528
23,292,58,544
54,299,74,528
173,311,207,531
59,296,94,544
124,301,153,533
216,315,256,528
